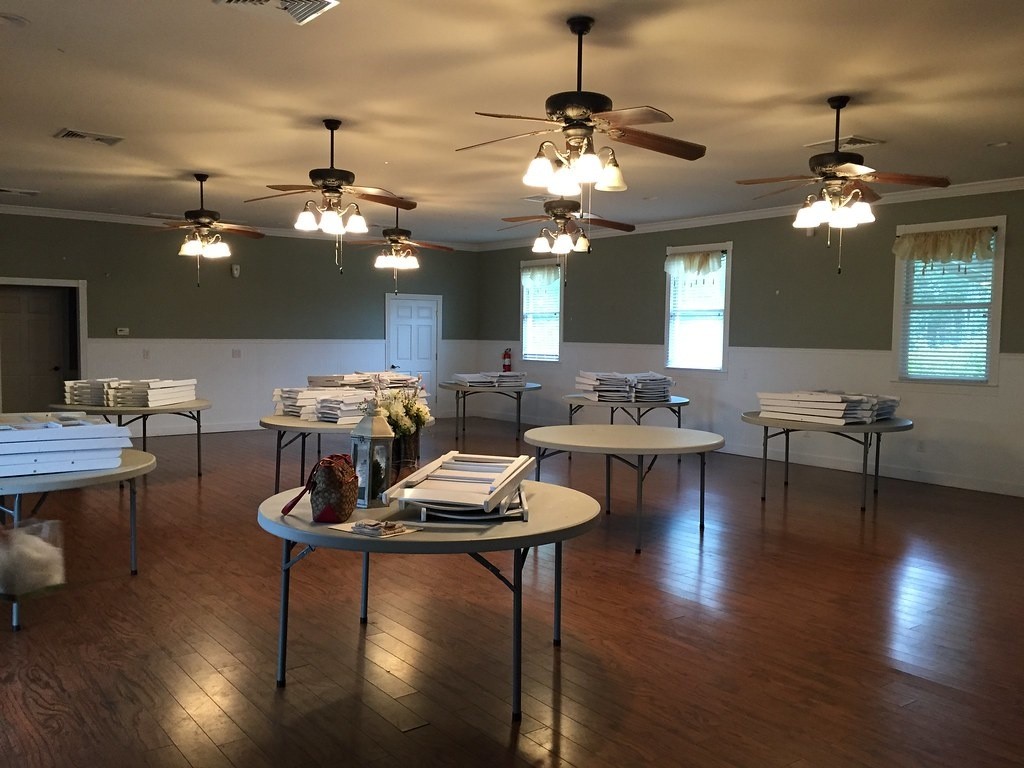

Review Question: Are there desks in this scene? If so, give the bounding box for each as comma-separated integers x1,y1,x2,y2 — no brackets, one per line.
259,415,435,495
0,448,159,632
562,393,692,467
50,401,215,484
739,412,916,514
523,424,726,554
439,380,542,442
255,478,604,725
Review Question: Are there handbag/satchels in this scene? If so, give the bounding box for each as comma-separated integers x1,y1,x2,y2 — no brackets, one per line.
280,453,359,523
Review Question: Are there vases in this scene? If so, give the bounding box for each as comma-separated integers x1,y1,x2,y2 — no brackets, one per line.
390,426,421,485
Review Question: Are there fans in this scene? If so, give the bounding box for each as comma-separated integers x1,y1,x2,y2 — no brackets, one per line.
244,119,418,211
497,195,636,235
454,17,707,162
734,95,952,201
130,173,268,240
351,198,454,254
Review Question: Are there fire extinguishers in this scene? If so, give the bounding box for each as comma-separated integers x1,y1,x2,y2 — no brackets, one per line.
503,347,512,372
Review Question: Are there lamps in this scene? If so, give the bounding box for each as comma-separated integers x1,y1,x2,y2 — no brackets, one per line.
293,200,370,235
177,230,232,260
522,139,629,199
533,226,592,255
372,245,421,270
790,190,876,229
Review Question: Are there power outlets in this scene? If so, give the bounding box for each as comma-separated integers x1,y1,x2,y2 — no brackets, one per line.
143,348,151,360
915,440,926,452
232,348,242,358
802,431,813,440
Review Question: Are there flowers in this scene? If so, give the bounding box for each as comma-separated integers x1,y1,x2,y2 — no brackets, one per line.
354,370,431,438
